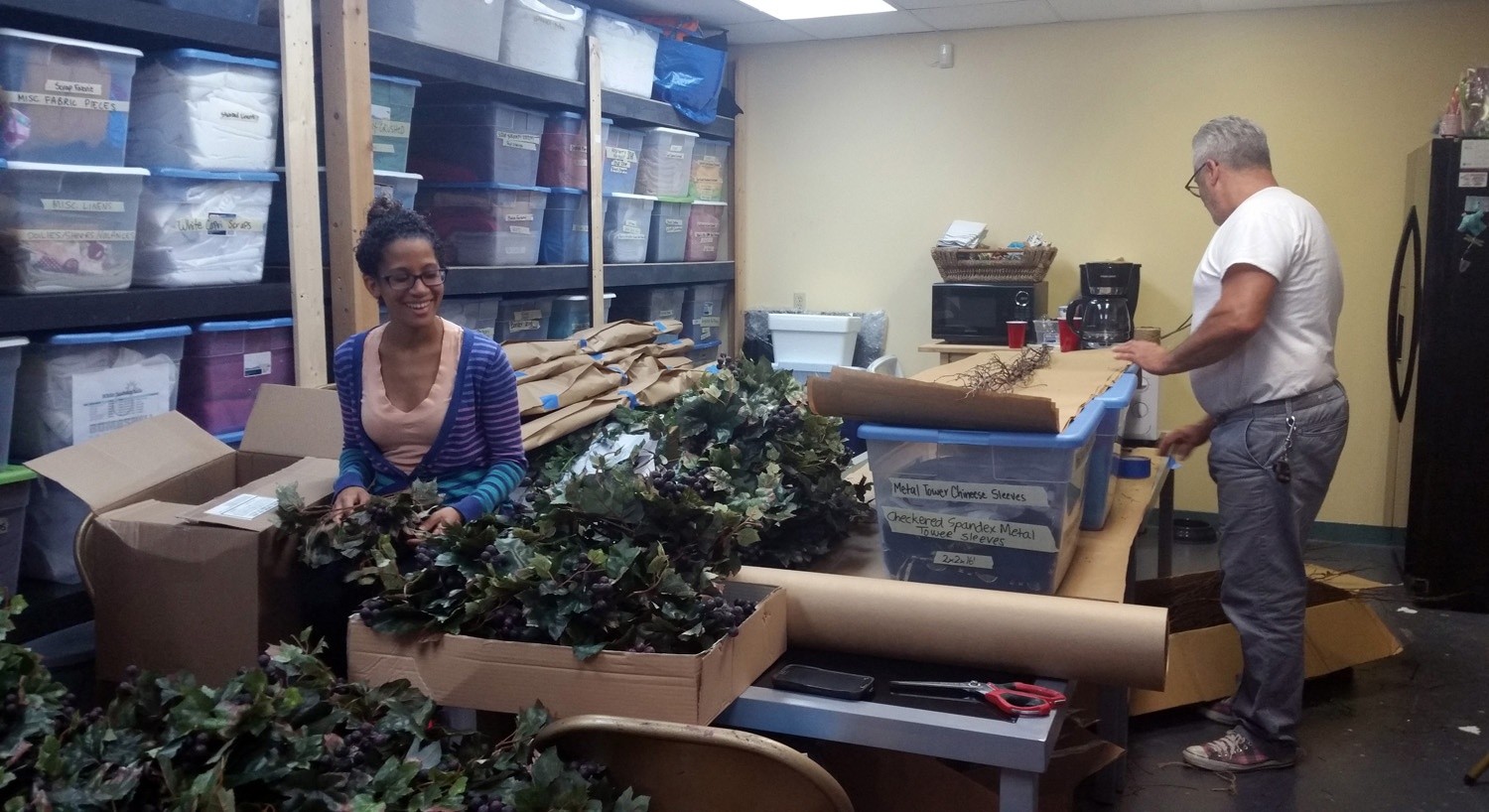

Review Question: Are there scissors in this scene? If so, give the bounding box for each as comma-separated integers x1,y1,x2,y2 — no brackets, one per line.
890,679,1067,717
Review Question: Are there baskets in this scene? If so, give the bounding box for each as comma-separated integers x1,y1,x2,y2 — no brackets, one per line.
931,245,1058,283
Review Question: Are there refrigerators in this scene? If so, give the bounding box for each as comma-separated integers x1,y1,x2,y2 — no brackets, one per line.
1387,136,1489,611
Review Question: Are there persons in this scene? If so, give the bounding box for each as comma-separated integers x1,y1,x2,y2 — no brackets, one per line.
1110,114,1351,772
298,199,530,684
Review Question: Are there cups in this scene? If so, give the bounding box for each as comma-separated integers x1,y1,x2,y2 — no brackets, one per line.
1056,317,1084,352
1006,321,1028,348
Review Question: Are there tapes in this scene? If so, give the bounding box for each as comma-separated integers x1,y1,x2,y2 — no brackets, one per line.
1120,455,1151,478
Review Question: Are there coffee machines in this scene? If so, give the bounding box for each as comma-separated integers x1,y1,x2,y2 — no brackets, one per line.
1067,262,1141,350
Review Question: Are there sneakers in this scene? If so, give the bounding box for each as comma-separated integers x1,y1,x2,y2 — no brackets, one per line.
1183,730,1297,773
1203,695,1235,723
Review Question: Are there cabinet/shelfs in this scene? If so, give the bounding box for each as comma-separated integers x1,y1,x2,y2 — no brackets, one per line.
323,0,746,642
0,0,329,732
1384,138,1489,610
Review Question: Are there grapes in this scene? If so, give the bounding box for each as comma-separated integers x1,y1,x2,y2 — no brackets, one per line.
0,662,613,812
359,351,803,653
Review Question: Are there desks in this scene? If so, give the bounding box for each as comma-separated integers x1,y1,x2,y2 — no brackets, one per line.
705,431,1176,812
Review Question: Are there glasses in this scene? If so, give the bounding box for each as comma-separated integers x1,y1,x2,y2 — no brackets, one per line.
372,267,446,291
1185,161,1220,198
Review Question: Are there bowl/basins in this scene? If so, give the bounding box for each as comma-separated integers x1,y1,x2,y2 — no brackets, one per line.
1174,518,1217,543
1058,306,1068,317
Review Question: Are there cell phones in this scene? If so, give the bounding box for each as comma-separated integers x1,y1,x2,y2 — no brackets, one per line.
771,664,875,700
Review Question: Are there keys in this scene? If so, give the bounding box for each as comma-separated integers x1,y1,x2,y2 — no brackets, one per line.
1273,461,1292,484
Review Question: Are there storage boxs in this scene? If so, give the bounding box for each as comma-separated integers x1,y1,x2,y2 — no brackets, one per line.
0,0,1401,812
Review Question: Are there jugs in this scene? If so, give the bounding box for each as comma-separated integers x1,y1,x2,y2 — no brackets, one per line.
1067,298,1130,344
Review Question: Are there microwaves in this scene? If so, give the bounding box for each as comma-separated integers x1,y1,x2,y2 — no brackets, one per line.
932,281,1041,343
